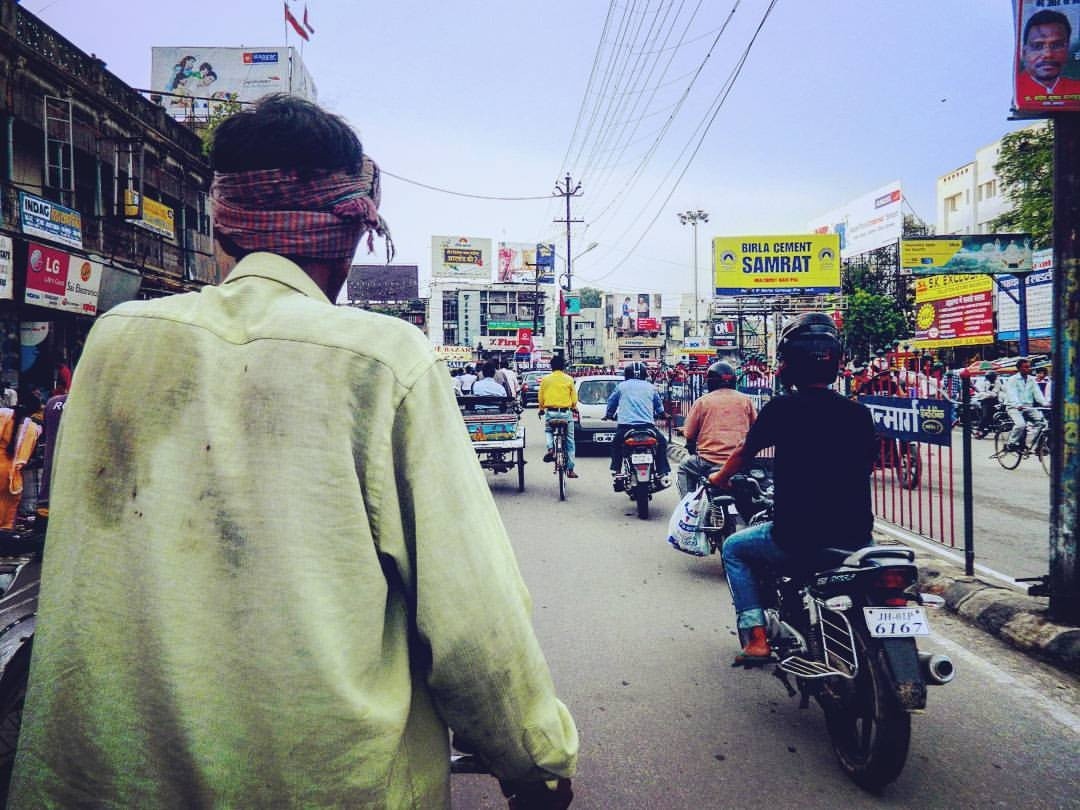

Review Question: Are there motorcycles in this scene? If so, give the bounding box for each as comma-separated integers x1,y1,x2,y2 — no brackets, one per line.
604,414,672,518
720,475,955,791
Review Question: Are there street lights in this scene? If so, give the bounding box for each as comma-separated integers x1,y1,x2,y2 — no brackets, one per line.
552,172,584,363
677,208,710,337
567,241,600,367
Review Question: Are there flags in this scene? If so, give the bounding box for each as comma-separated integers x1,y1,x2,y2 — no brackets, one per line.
284,4,309,40
304,7,314,33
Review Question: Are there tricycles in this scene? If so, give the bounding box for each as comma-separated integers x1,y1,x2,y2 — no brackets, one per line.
455,395,528,492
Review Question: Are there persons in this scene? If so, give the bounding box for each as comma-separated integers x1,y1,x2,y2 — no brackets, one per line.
449,358,521,409
0,336,85,533
846,363,900,466
1014,10,1080,111
166,55,217,104
677,362,757,498
707,310,877,669
637,297,649,312
901,359,1052,428
622,297,635,329
605,363,672,476
1004,359,1051,452
7,89,580,810
537,356,578,478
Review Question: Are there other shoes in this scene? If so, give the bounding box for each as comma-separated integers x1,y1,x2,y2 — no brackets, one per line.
544,448,553,462
569,473,578,478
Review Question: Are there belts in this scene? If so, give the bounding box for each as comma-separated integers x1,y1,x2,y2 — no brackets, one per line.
548,408,569,412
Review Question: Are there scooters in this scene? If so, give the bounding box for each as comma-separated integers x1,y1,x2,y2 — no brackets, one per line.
672,426,745,552
874,437,922,490
966,398,1015,438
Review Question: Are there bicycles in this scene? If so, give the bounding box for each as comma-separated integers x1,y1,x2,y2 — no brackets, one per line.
550,418,571,500
992,406,1052,477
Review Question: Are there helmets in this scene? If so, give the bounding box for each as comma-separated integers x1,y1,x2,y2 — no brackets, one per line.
774,312,841,385
625,363,649,380
706,362,737,392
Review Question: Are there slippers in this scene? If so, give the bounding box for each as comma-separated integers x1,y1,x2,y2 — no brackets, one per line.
731,650,776,668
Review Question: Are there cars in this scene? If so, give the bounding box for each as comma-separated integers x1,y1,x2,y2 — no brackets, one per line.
520,375,544,408
574,375,627,445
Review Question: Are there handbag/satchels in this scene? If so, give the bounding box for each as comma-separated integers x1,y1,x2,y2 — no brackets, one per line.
667,485,713,558
